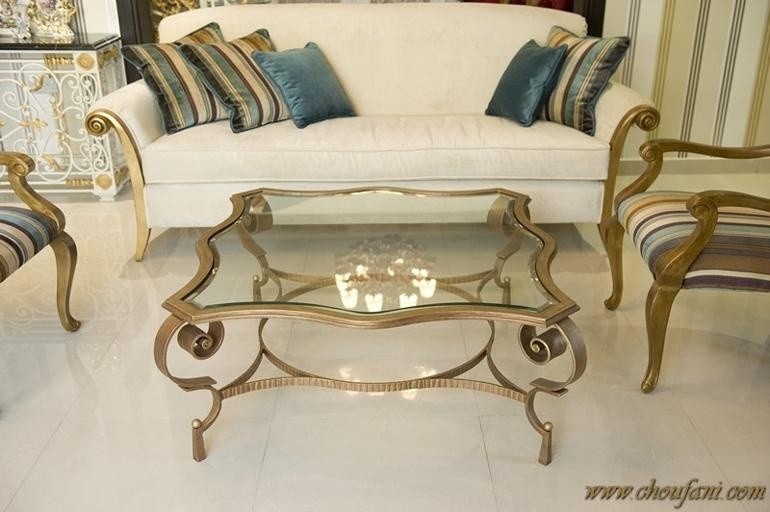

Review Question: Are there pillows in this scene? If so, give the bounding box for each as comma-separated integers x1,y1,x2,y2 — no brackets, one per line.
120,22,232,134
485,39,568,127
538,26,629,137
177,28,291,134
250,41,358,128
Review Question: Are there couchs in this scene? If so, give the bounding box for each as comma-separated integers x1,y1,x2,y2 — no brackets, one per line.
85,1,661,262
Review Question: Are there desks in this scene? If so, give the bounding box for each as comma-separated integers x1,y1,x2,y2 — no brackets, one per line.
0,33,130,202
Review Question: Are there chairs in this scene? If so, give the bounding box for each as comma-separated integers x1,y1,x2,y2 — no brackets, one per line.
0,150,82,332
605,140,770,392
